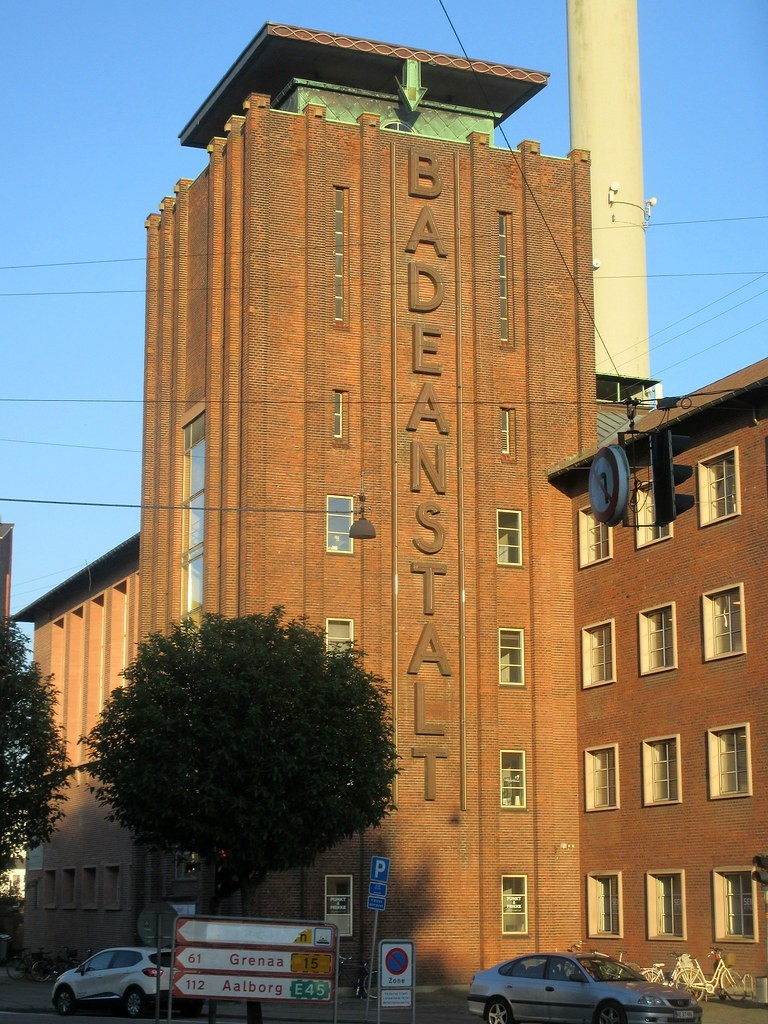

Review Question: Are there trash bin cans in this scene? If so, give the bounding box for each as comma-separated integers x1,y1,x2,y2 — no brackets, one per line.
755,976,768,1004
0,935,12,963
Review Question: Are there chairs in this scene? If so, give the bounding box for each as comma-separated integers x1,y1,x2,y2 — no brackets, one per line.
551,965,559,979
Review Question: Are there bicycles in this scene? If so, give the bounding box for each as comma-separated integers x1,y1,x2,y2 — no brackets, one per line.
554,946,746,1003
7,945,92,984
334,955,379,998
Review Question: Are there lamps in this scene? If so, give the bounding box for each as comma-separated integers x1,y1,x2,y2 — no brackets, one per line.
347,513,377,540
644,197,657,208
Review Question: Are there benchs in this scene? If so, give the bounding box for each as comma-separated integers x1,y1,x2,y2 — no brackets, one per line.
512,966,544,979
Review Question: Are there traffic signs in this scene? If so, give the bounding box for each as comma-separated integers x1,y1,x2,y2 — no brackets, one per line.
172,920,334,1002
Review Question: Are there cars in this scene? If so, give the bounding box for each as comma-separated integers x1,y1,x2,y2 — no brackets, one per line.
52,946,204,1018
468,951,703,1024
